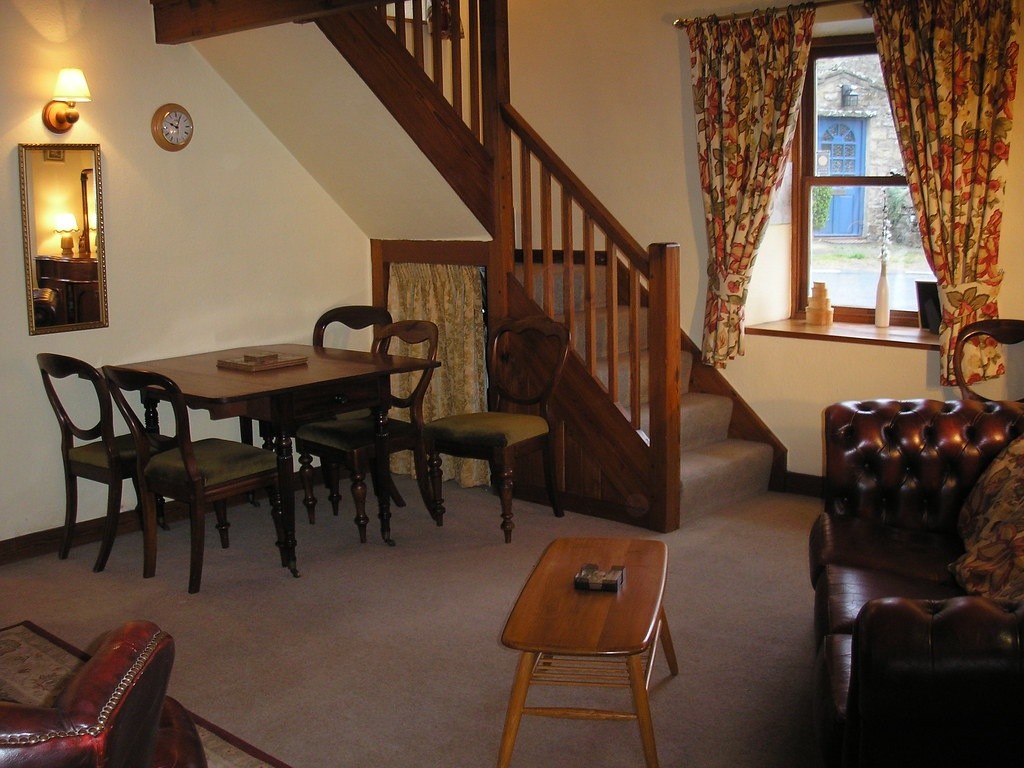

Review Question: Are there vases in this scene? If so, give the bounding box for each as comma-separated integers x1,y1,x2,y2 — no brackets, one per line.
874,260,890,329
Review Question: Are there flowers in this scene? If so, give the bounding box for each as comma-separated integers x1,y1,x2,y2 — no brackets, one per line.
878,188,891,260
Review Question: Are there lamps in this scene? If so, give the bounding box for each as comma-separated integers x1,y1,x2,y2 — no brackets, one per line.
44,70,91,134
54,215,78,257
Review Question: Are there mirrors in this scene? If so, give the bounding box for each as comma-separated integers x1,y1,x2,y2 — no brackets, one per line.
81,170,98,259
16,142,111,335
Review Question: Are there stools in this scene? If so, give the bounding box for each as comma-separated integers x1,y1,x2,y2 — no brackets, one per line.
497,539,669,768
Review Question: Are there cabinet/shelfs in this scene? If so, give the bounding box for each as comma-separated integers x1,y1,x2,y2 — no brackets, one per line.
36,260,98,324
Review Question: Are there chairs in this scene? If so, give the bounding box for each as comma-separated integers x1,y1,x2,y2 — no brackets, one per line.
37,353,231,573
261,306,394,454
101,363,296,592
301,320,437,546
418,318,571,538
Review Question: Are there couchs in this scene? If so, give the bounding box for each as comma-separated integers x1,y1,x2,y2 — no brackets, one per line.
0,621,210,768
809,397,1024,768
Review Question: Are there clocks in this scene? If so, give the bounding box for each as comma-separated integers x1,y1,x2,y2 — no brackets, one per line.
151,102,193,151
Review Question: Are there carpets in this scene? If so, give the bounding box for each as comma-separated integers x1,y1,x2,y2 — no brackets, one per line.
0,601,289,768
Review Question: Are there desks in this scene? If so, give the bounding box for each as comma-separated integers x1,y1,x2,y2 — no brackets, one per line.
110,342,442,565
